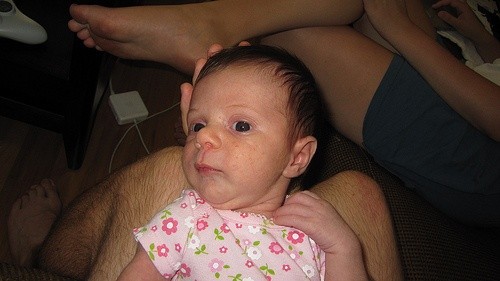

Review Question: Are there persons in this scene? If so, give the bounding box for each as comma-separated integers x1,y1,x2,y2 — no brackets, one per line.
7,147,402,280
116,46,368,280
67,0,500,240
